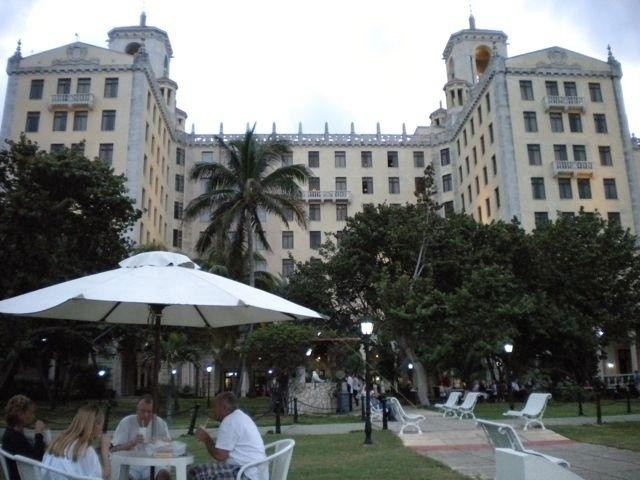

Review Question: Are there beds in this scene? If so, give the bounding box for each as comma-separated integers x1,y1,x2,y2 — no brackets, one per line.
1,249,330,480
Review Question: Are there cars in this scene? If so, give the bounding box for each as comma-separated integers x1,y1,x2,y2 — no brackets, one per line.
195,425,208,442
42,428,52,447
138,427,148,444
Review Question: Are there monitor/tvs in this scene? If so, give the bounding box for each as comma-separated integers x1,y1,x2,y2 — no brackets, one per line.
153,449,173,458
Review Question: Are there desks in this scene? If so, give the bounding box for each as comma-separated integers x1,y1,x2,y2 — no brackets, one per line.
360,321,375,443
504,343,515,412
206,367,213,408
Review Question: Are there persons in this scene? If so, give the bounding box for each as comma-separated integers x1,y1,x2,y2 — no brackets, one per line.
399,373,553,406
184,390,271,480
2,394,49,480
108,393,173,480
304,368,399,423
42,403,112,480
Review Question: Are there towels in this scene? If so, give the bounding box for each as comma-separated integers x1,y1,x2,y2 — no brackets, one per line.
431,387,439,402
410,390,417,405
337,393,350,413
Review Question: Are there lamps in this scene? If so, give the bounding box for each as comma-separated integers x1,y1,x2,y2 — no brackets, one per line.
236,438,297,480
0,442,103,480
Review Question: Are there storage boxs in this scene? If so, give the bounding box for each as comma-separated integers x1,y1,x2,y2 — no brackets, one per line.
364,391,572,469
